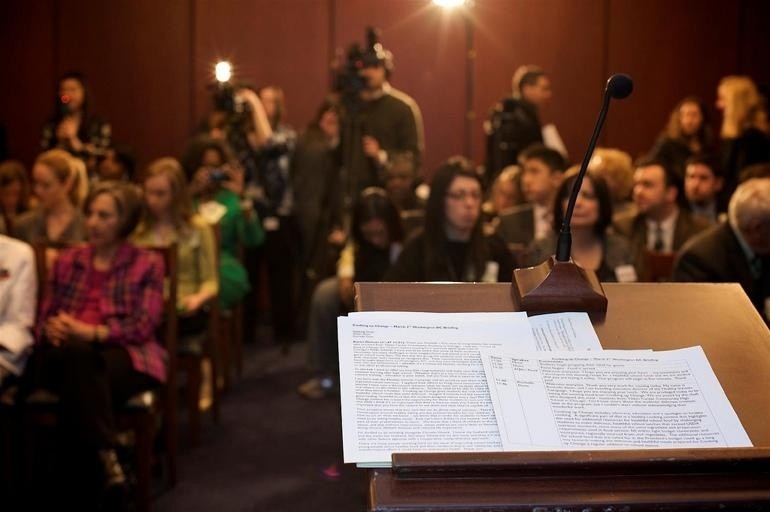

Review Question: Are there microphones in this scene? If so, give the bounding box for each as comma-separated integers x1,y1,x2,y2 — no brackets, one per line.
555,71,636,266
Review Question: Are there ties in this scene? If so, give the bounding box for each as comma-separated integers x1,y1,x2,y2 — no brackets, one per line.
655,226,663,253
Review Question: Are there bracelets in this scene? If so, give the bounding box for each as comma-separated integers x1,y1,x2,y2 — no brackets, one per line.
93,322,102,347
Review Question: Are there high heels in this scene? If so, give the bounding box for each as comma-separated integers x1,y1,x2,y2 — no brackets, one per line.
101,452,136,489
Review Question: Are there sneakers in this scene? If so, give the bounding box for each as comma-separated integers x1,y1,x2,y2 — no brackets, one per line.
297,373,338,397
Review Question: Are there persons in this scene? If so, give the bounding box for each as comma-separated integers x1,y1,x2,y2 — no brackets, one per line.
1,31,770,400
0,233,41,403
40,180,170,491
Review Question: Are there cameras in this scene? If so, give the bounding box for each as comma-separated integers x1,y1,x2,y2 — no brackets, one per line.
203,81,249,118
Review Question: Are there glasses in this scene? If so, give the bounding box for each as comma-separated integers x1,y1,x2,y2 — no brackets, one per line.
447,187,482,200
